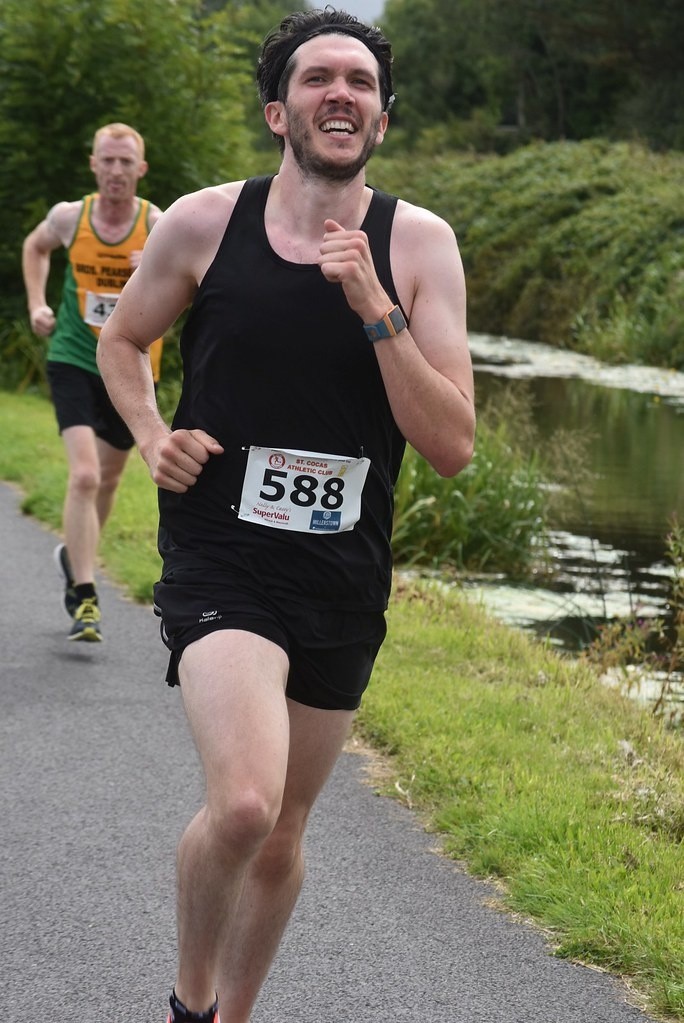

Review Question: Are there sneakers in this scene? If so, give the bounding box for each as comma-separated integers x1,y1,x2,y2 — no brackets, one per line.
66,596,103,643
52,543,76,621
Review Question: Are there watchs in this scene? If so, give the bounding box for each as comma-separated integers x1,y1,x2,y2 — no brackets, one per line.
363,304,407,342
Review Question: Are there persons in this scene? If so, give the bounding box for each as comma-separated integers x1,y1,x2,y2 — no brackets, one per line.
21,122,165,641
95,4,478,1023
